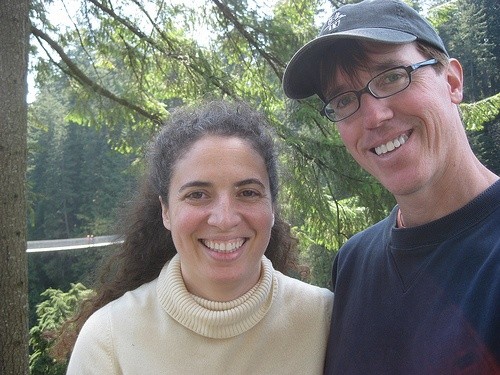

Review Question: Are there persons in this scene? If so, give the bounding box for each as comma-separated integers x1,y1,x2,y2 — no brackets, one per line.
39,97,336,374
280,0,499,375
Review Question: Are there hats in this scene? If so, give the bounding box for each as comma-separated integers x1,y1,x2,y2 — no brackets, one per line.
281,0,450,100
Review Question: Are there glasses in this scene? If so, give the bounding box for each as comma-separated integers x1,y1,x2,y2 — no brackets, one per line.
318,57,443,122
